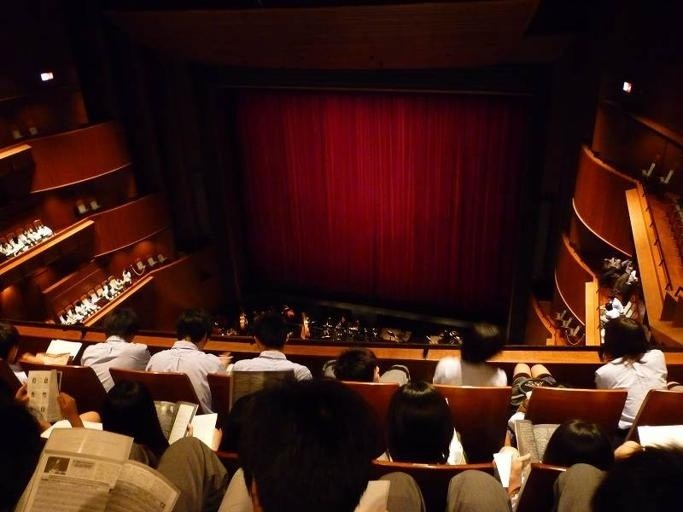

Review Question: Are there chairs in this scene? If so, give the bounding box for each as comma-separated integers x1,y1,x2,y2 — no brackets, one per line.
624,389,682,441
208,372,231,417
56,275,117,323
0,219,47,259
524,384,627,428
430,382,513,452
370,458,495,512
108,366,204,416
513,464,567,512
341,380,401,421
18,359,106,408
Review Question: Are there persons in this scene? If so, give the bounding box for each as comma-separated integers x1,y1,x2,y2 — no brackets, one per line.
1,258,683,511
0,224,54,256
